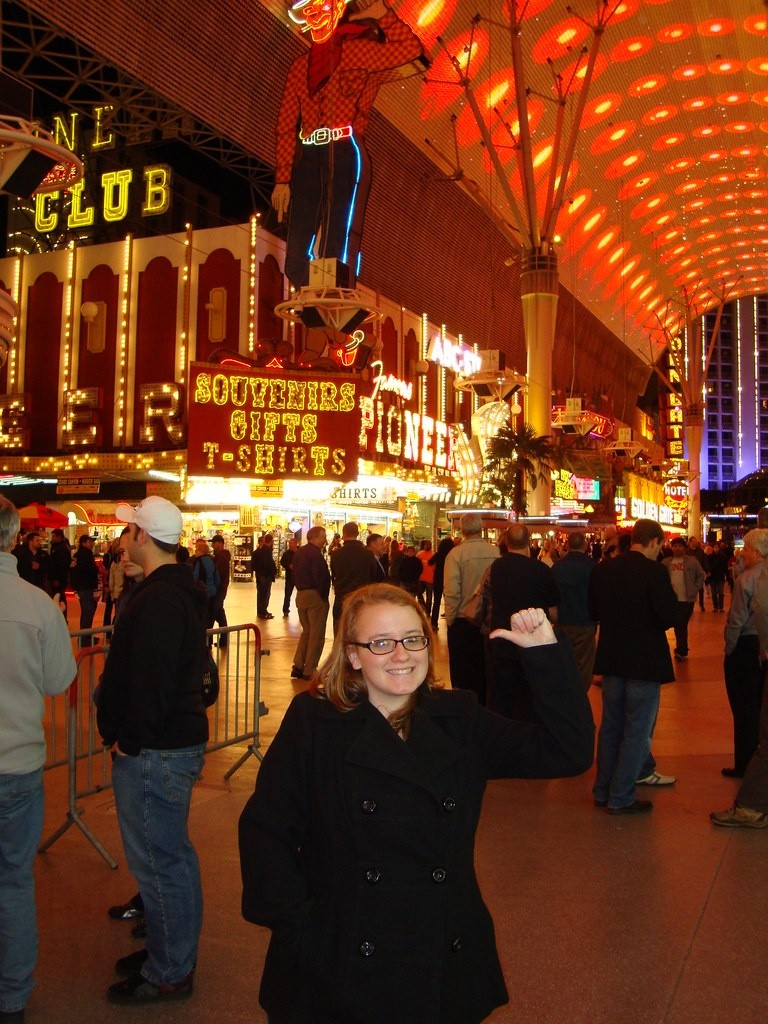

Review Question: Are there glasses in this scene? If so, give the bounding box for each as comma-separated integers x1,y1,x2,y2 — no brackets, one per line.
351,635,429,655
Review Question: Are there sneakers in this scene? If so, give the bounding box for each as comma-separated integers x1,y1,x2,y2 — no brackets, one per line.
709,803,768,829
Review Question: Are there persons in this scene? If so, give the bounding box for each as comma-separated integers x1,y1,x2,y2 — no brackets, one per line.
96,495,209,1007
0,492,77,1024
236,583,596,1024
0,513,768,829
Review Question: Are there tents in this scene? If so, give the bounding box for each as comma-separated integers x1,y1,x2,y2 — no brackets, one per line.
14,502,69,533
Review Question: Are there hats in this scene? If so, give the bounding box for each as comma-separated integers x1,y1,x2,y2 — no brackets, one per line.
208,535,224,542
79,534,96,543
115,496,183,544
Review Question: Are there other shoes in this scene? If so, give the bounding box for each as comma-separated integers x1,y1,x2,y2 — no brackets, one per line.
721,766,742,778
107,974,193,1004
634,771,677,786
116,949,149,976
108,901,144,919
132,919,147,938
609,800,653,813
291,666,303,677
